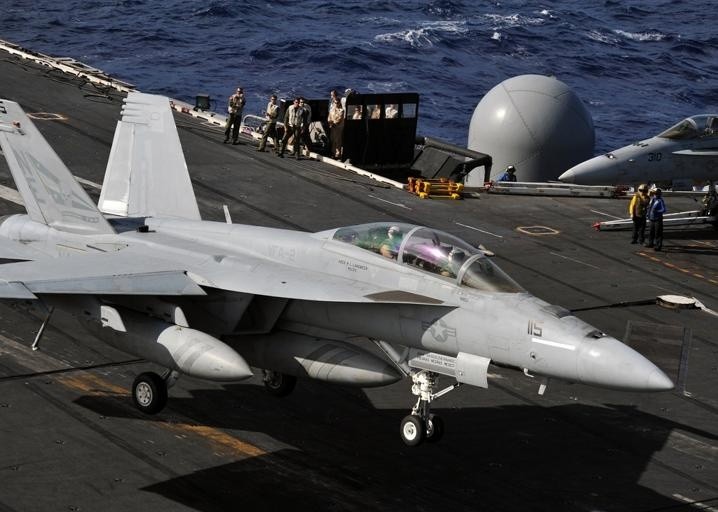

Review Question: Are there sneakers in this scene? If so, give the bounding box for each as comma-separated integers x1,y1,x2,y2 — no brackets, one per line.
632,238,662,252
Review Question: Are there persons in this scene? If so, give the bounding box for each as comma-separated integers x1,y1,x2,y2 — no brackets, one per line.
645,188,667,253
497,164,518,181
223,86,246,145
627,184,651,247
253,87,399,163
438,248,465,279
377,225,411,262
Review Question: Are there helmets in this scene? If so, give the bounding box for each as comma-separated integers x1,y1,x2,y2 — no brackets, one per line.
638,184,648,195
506,165,516,172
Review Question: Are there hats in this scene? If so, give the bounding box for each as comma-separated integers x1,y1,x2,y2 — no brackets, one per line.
651,188,662,194
237,88,243,92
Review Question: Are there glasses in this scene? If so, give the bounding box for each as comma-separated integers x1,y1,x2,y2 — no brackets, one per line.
335,102,339,104
272,99,276,100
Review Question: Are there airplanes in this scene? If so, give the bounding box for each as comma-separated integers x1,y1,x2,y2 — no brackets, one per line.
3,88,677,448
558,109,717,193
